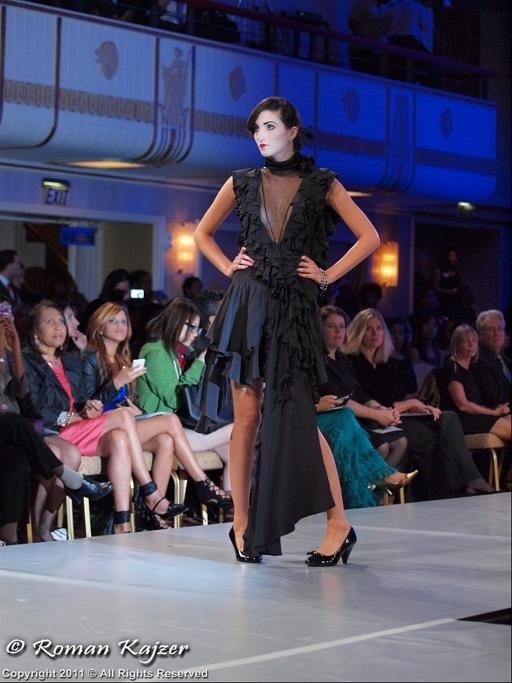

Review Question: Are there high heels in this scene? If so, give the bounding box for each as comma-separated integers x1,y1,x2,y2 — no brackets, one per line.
67,468,418,564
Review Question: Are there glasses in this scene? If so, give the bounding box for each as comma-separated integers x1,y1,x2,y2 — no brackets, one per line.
184,323,203,334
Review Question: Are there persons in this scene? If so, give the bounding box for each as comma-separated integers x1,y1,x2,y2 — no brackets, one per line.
1,248,512,547
190,95,384,567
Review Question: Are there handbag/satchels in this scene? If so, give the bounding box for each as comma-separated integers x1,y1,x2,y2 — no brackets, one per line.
175,385,202,422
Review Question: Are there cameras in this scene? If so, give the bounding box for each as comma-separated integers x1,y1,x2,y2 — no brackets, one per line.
113,287,146,310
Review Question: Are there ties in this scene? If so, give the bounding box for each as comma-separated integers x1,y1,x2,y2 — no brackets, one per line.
496,354,512,382
6,283,14,300
